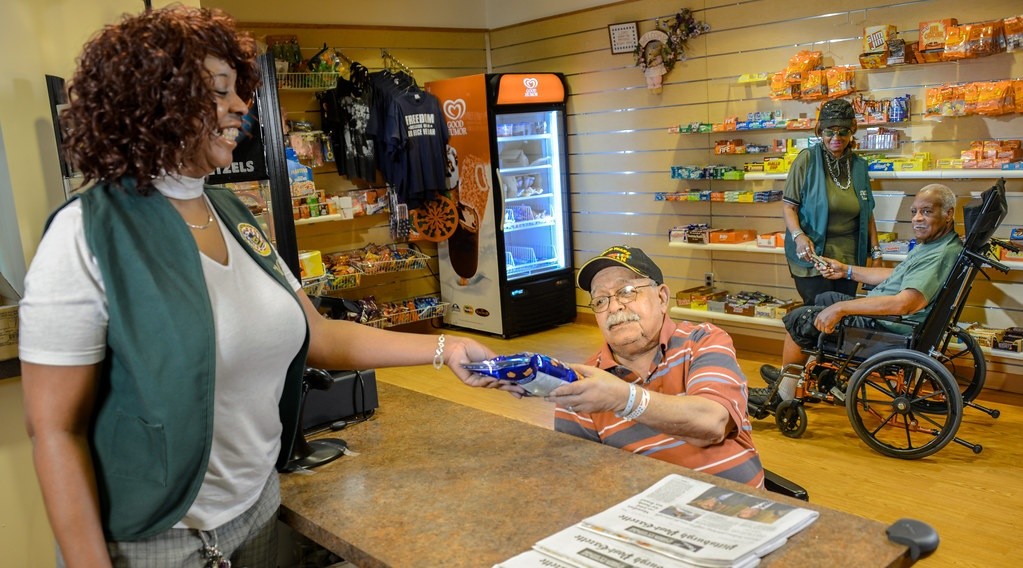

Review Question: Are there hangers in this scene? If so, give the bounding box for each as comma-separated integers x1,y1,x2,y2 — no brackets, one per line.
343,52,416,98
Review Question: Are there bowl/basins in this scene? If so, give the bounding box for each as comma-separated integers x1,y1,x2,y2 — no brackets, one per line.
496,121,548,137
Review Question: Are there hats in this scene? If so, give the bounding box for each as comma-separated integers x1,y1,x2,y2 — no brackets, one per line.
819,99,855,129
577,245,664,291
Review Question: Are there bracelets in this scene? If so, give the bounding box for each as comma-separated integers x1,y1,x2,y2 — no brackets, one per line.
791,229,805,243
625,387,650,419
615,382,636,418
847,265,852,279
871,246,882,260
433,333,445,371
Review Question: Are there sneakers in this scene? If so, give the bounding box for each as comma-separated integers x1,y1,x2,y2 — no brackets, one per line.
760,364,781,386
748,387,789,416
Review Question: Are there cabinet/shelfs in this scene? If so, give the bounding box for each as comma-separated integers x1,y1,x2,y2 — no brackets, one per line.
654,41,1023,378
204,48,445,331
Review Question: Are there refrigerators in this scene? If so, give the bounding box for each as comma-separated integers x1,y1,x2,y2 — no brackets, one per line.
424,73,578,340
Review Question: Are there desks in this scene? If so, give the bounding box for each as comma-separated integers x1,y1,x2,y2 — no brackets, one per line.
279,378,940,568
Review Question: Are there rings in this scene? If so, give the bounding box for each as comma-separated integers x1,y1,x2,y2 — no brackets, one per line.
796,247,810,259
568,406,573,411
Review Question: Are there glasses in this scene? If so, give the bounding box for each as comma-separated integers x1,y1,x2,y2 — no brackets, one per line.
587,283,659,314
822,130,850,136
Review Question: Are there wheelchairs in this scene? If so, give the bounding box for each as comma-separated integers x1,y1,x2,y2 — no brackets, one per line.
746,176,1021,461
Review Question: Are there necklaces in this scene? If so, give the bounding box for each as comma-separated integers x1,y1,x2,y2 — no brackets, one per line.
184,194,214,230
825,150,851,191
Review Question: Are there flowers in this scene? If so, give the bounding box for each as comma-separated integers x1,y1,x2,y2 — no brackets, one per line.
631,7,710,72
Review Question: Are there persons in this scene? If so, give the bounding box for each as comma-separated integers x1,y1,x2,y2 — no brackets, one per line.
748,184,965,416
19,3,524,568
549,246,765,493
782,99,882,403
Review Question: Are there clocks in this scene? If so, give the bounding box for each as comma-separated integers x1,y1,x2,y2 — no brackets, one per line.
607,21,640,54
